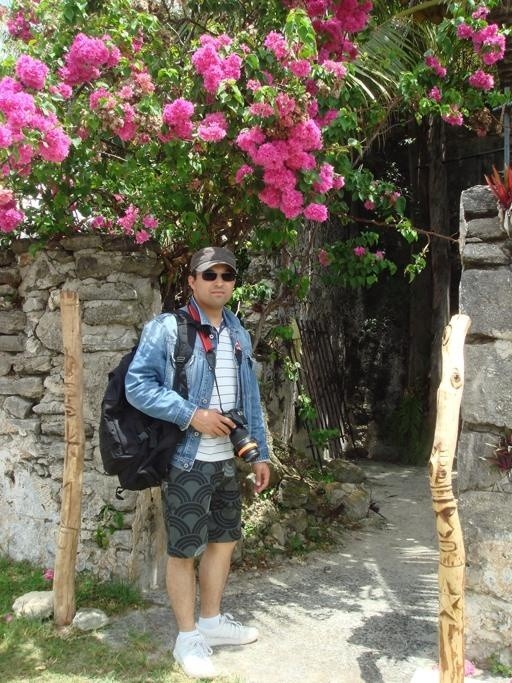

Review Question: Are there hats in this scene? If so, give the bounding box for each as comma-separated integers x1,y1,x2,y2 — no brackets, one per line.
189,246,237,274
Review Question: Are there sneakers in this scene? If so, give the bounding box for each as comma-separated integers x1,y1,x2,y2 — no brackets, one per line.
195,615,258,647
172,633,219,679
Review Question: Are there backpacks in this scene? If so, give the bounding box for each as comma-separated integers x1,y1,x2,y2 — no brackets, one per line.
99,309,198,490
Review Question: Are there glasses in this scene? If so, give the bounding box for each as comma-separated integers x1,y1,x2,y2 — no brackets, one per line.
196,272,237,281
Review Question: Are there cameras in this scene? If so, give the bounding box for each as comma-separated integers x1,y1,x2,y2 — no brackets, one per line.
220,408,260,464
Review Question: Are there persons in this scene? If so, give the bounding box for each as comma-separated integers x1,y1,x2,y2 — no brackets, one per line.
124,248,272,681
